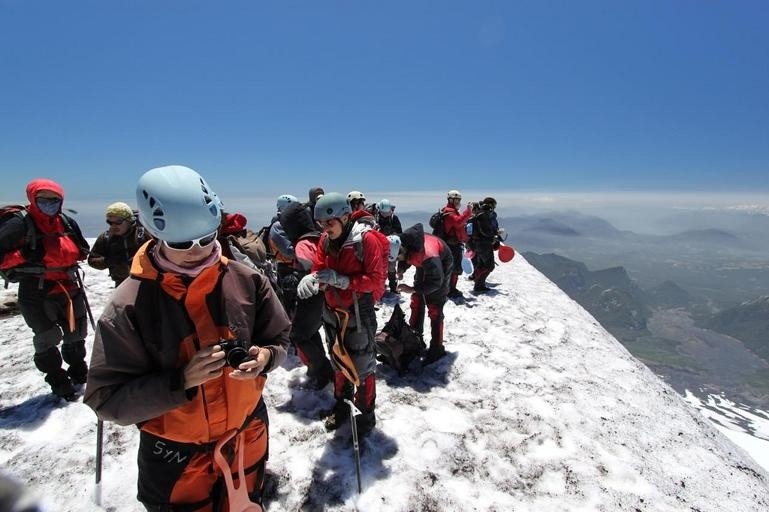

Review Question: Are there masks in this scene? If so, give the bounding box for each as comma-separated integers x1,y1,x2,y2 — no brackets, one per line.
36,203,61,216
456,202,461,209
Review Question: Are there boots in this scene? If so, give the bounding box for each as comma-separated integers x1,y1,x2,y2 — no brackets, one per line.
469,256,480,280
447,275,462,298
64,342,90,386
352,377,378,441
295,342,336,393
386,271,404,294
32,348,79,402
472,269,492,292
324,382,353,429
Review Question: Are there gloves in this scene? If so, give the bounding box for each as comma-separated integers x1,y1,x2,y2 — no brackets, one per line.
315,268,350,290
296,273,318,300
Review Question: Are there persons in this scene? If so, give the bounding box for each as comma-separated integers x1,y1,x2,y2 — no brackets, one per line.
430,190,473,299
294,192,385,459
364,201,379,225
0,180,90,401
231,213,267,268
280,203,332,392
88,199,155,292
218,213,264,274
304,184,325,230
82,165,292,512
395,223,454,366
371,200,402,295
347,189,381,232
268,194,295,323
464,198,504,292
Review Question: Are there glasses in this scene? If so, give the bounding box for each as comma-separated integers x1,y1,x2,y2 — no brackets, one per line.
34,196,60,205
315,215,339,229
161,229,218,251
106,217,126,226
455,199,461,204
355,202,365,207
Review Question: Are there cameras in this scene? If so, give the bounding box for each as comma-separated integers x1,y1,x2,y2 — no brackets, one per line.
216,339,250,369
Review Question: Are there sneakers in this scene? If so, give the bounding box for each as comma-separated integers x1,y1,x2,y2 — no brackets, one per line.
305,364,333,394
404,335,424,348
422,342,445,365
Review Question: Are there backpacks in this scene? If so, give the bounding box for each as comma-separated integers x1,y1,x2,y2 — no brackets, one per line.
103,209,144,255
321,210,390,333
374,303,423,370
430,207,455,242
0,203,87,289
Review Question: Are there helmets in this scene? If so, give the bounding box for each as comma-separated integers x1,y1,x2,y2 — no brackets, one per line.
275,191,298,208
446,189,462,200
314,193,353,219
378,200,392,213
345,190,365,204
135,165,224,242
386,234,402,263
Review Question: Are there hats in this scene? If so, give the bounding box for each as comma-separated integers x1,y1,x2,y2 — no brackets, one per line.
24,180,66,237
104,201,132,222
280,199,312,242
310,186,323,200
222,213,248,233
